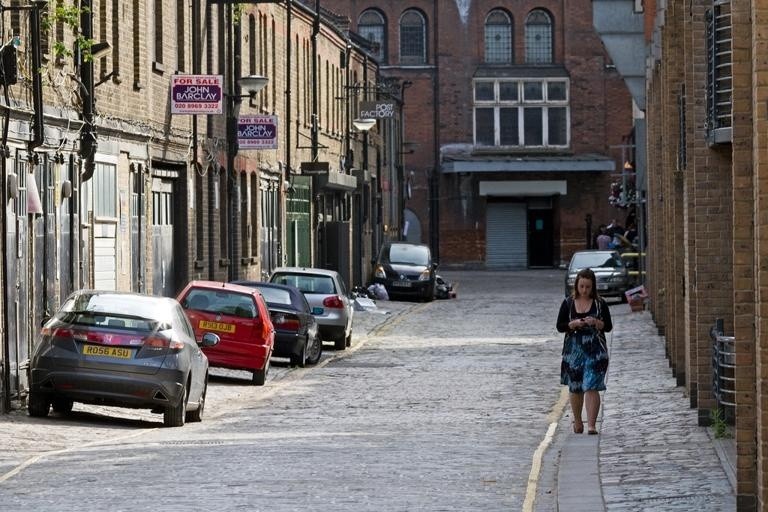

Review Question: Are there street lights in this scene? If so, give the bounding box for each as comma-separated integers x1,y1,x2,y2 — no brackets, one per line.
353,115,376,275
223,74,269,280
399,78,413,239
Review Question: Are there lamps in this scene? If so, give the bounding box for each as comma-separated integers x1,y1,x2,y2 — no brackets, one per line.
73,38,113,67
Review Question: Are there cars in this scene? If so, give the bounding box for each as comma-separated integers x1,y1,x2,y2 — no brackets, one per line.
228,281,323,367
170,264,275,386
621,252,646,282
564,247,631,305
28,290,221,426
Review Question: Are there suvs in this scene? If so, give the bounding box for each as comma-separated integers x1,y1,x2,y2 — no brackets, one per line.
373,241,439,301
264,264,354,350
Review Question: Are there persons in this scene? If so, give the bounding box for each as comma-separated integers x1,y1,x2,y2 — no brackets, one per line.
557,268,614,435
595,218,637,252
